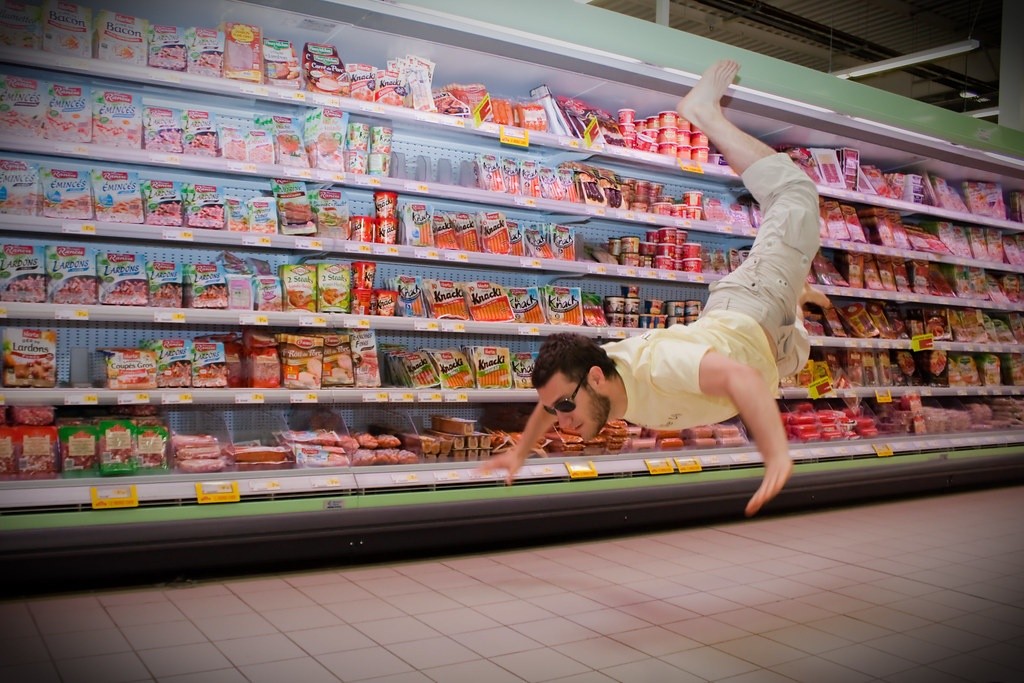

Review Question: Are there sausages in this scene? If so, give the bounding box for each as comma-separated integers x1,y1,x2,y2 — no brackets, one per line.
387,91,609,389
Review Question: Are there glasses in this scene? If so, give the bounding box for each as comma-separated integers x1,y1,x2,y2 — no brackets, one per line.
543,374,586,415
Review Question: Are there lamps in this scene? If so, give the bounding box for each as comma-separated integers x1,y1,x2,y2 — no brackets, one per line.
832,39,979,80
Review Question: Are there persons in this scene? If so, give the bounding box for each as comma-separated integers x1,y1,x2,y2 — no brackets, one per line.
476,58,821,514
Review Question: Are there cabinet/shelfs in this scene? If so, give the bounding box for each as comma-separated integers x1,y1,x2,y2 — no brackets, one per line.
0,0,1023,513
